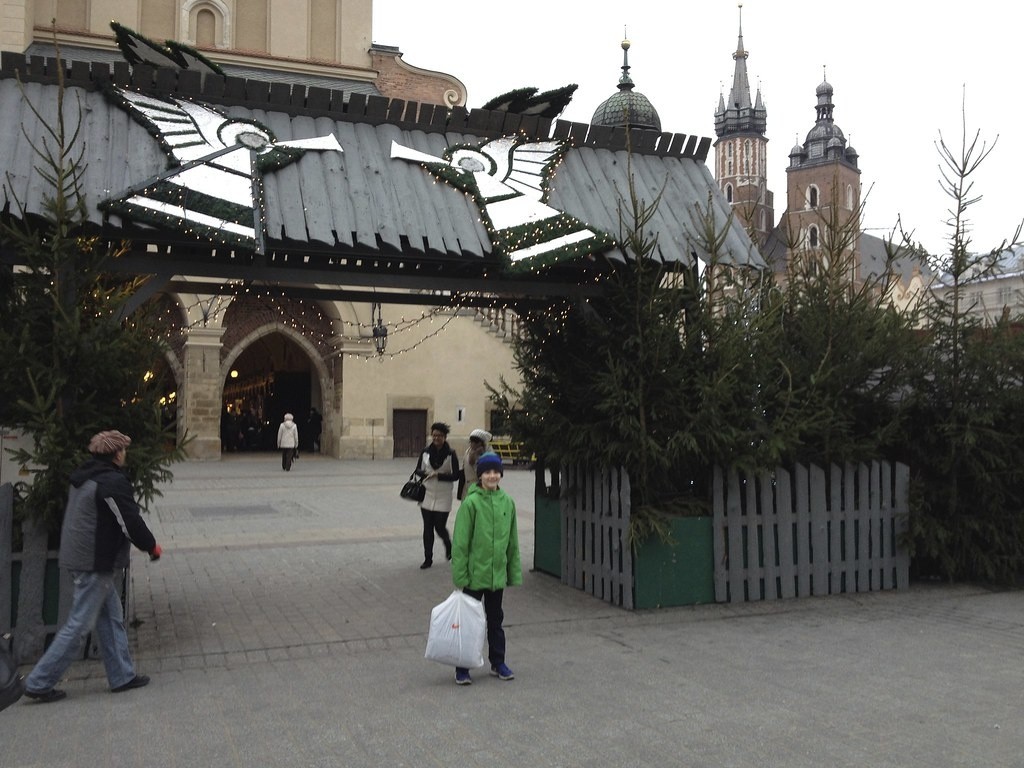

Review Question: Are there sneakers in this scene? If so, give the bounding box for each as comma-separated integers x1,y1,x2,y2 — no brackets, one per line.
455,673,471,684
491,665,514,680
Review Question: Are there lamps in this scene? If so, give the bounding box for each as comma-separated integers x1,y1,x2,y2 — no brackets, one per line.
372,302,387,357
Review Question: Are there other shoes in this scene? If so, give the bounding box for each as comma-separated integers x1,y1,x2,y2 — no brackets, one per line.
421,559,432,569
446,545,451,560
112,675,150,693
25,688,66,702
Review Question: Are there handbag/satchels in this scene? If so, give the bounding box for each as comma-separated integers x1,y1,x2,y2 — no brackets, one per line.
425,588,487,668
400,469,426,502
293,447,299,459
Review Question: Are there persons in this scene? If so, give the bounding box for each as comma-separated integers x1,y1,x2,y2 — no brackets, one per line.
451,453,523,684
24,431,162,702
278,413,299,469
222,405,324,453
457,430,496,502
414,422,459,568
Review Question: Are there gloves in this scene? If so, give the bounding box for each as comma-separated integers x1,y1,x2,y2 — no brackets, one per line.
147,545,161,561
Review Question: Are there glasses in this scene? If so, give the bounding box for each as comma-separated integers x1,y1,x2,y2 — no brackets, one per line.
430,433,445,438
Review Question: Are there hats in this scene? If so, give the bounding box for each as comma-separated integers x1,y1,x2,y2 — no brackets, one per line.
88,430,132,455
470,429,492,445
476,452,503,478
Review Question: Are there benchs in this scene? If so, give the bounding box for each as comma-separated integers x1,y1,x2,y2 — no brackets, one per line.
490,441,537,472
